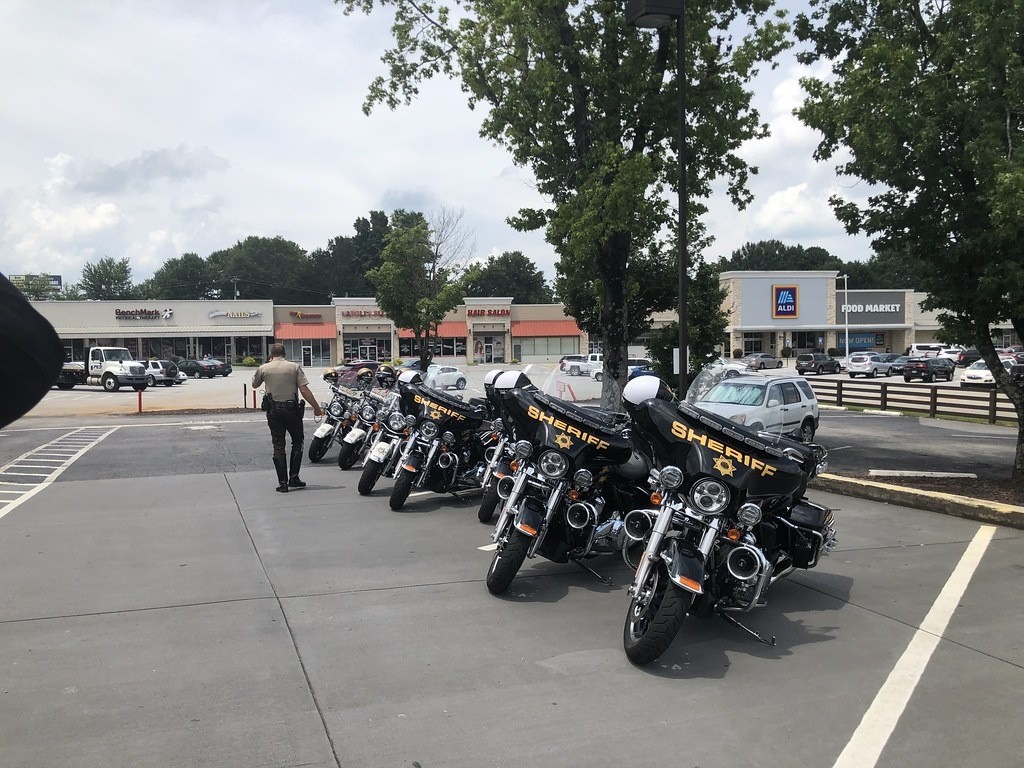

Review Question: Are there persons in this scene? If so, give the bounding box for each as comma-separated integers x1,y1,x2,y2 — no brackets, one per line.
494,342,504,356
474,341,483,353
204,355,208,361
886,345,890,353
207,352,213,360
174,356,179,362
252,342,322,493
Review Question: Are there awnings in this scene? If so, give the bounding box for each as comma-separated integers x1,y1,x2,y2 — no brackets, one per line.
398,320,468,338
511,320,581,337
274,322,337,340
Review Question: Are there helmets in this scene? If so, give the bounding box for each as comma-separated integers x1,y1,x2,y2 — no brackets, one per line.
397,367,412,378
357,368,373,389
483,369,504,395
399,371,422,390
323,369,337,384
494,370,533,398
376,364,395,387
621,375,676,420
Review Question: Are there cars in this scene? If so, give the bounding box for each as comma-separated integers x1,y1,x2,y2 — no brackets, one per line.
703,355,752,379
628,366,660,381
173,370,188,385
175,360,219,379
394,360,435,372
208,359,232,377
877,353,903,363
890,356,921,374
959,343,1024,387
337,361,385,386
840,352,878,367
422,364,468,390
741,353,784,369
936,347,964,363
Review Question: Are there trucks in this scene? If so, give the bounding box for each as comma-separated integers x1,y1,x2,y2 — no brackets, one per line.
53,346,151,394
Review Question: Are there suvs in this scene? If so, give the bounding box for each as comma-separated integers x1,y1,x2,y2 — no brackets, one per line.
904,358,954,383
794,353,841,375
559,354,590,377
590,358,654,382
693,374,822,446
138,360,180,388
848,355,893,377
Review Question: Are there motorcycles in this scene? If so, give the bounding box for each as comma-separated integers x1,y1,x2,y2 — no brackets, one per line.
622,363,837,663
308,363,650,596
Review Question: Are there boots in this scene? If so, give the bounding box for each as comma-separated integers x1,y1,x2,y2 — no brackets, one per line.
288,451,306,488
273,454,289,493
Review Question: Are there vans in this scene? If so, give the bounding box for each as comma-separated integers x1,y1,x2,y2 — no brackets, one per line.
587,354,604,373
908,343,968,358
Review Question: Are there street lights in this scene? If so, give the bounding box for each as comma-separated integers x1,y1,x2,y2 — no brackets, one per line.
834,274,849,371
